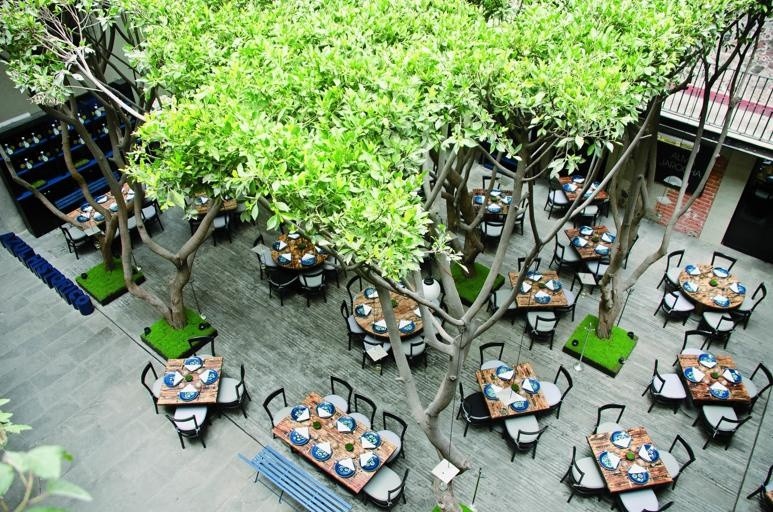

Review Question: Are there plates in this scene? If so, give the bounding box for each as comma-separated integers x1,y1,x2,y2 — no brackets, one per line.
572,174,582,179
364,288,375,298
521,378,540,394
316,402,334,417
712,266,729,278
184,356,202,366
683,282,698,293
520,287,523,294
273,241,279,251
723,368,741,384
644,445,658,463
94,215,104,221
200,369,217,385
545,280,560,292
179,392,198,401
714,301,729,307
610,431,631,449
578,225,592,232
605,232,615,242
709,389,729,400
335,463,355,478
487,208,501,212
562,183,575,192
372,325,387,333
698,353,716,369
484,384,496,400
684,265,695,274
290,430,310,446
358,455,379,471
355,305,371,317
490,189,500,191
599,453,619,470
596,249,608,255
527,269,541,277
397,323,414,333
81,202,90,210
736,284,744,294
336,415,355,433
570,236,579,247
683,367,695,383
301,258,315,265
534,297,549,304
627,467,649,486
507,196,512,204
96,195,104,201
475,195,481,204
496,365,514,381
510,401,529,411
362,432,380,447
77,216,89,222
163,372,173,386
278,256,289,265
290,405,310,420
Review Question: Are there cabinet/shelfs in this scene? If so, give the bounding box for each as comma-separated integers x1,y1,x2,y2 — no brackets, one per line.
1,98,141,240
